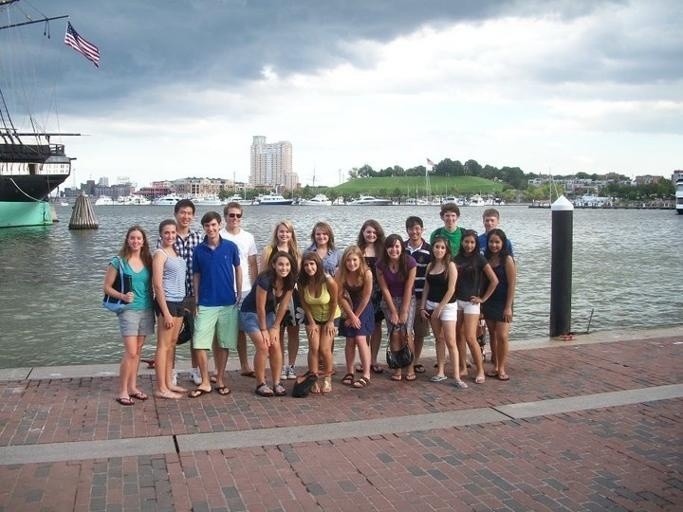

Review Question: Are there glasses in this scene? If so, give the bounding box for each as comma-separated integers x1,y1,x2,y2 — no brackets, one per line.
227,212,243,219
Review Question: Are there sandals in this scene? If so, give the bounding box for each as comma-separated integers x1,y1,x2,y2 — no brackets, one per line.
311,376,332,393
155,384,189,400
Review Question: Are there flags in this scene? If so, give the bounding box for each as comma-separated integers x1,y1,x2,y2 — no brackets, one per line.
63,21,100,69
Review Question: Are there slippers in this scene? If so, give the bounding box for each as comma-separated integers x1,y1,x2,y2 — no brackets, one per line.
317,359,472,378
391,373,402,382
450,379,468,388
210,371,219,383
430,375,448,382
115,396,135,406
215,384,232,395
405,373,417,381
351,375,372,387
240,369,257,379
475,375,486,384
254,382,274,396
498,371,510,381
129,391,149,400
341,372,354,386
487,367,499,377
273,383,286,397
190,388,214,397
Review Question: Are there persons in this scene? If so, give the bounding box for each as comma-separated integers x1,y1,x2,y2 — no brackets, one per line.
210,202,266,383
156,199,203,386
103,225,153,405
188,211,243,398
152,219,189,399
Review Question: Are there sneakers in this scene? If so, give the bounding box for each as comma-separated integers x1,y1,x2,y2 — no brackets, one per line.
280,364,288,380
188,367,202,385
171,367,177,385
287,364,296,379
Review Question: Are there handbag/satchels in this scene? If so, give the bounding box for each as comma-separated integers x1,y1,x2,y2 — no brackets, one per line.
175,307,194,346
292,367,337,398
385,321,414,369
102,256,132,315
340,288,354,319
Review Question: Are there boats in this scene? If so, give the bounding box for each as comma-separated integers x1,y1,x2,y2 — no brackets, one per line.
674,177,682,216
0,0,91,228
